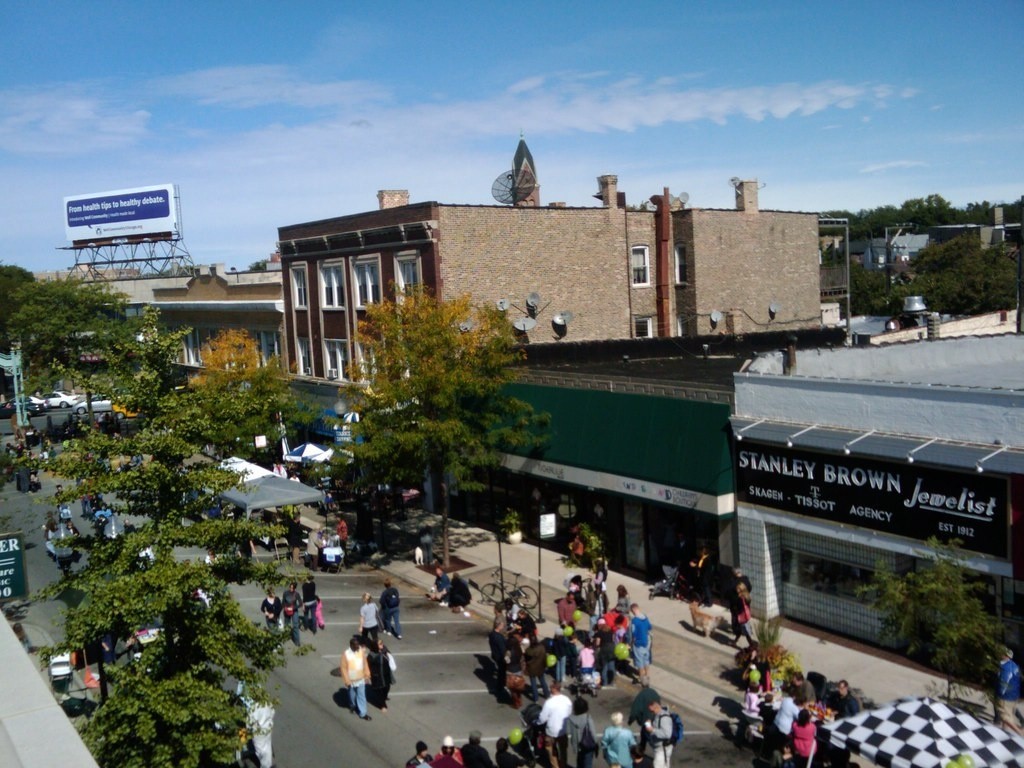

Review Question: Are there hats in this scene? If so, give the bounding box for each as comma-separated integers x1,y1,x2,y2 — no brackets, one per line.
443,735,454,747
1005,649,1013,658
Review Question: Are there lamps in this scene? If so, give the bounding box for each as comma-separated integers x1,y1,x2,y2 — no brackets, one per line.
976,444,1011,474
736,418,767,439
784,425,817,448
843,431,875,455
904,436,939,463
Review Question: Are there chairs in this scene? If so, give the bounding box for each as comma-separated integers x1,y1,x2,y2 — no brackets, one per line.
274,536,292,562
326,557,346,574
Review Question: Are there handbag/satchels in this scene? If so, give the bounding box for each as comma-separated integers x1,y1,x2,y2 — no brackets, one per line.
373,603,384,633
578,714,596,753
737,595,750,623
506,674,525,689
386,652,397,672
284,605,293,615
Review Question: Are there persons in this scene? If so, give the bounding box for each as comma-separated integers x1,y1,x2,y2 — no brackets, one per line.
260,573,325,648
339,579,402,720
3,412,258,667
245,690,276,768
264,460,349,573
993,646,1024,737
406,527,713,768
726,566,859,768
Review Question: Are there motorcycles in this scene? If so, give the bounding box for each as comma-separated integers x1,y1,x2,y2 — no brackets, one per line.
45,524,77,576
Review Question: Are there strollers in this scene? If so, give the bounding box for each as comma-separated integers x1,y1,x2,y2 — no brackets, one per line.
58,503,72,522
649,565,691,600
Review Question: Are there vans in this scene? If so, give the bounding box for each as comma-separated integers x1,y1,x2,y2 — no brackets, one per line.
48,652,72,680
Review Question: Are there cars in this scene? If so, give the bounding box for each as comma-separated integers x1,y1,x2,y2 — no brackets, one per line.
72,394,113,415
0,396,49,419
112,399,150,419
50,390,80,407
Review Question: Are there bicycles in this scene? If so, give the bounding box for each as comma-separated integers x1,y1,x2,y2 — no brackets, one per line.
481,566,539,609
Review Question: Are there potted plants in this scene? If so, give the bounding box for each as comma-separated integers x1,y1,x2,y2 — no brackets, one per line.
498,505,527,547
733,614,799,688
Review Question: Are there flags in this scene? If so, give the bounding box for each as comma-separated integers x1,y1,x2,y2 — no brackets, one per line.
281,422,292,461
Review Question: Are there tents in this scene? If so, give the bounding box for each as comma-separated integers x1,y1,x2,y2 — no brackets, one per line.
188,456,327,534
815,694,1024,768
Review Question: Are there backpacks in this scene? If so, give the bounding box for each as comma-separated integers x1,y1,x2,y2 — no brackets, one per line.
385,587,400,608
658,712,683,748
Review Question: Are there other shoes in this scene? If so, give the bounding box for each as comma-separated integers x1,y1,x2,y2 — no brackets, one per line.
398,635,402,639
361,715,372,720
384,696,390,700
439,601,449,606
383,703,387,709
383,630,391,636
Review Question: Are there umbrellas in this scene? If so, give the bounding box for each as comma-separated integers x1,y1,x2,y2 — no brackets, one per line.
343,411,359,424
284,441,335,468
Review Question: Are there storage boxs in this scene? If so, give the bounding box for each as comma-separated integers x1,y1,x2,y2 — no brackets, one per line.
322,546,346,565
